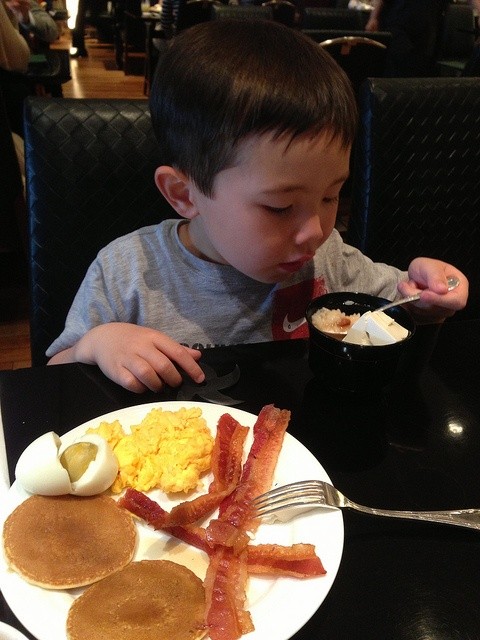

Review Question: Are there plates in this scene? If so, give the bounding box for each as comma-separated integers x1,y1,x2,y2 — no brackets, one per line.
0,401,345,640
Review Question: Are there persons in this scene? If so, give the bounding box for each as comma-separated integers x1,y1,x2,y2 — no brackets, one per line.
0,0,142,74
365,0,450,78
36,17,470,396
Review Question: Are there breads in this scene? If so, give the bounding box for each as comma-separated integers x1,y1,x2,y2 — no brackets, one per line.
66,561,208,639
3,493,141,588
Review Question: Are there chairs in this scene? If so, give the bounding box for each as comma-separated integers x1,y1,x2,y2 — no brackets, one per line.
23,96,182,366
354,76,479,263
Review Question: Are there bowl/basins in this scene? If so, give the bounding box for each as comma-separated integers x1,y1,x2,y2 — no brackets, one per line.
305,291,417,381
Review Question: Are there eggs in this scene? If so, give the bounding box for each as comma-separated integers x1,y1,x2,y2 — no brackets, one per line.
13,431,122,499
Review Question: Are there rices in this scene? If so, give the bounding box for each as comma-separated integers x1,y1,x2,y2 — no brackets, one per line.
312,311,358,334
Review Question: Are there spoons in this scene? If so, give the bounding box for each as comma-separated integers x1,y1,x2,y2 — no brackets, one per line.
313,274,460,335
250,479,480,531
